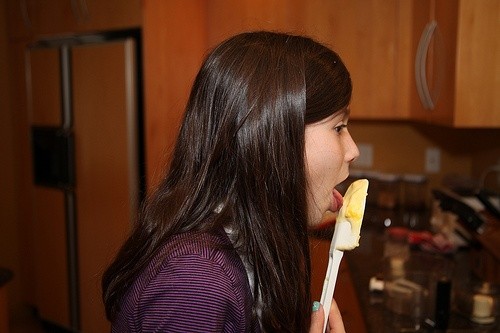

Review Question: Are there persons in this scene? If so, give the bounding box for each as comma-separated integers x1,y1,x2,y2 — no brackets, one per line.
102,30,359,333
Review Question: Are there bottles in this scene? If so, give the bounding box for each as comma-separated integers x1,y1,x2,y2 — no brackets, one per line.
429,278,453,333
385,228,416,284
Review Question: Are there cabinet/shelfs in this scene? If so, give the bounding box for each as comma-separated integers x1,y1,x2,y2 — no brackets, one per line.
203,0,500,128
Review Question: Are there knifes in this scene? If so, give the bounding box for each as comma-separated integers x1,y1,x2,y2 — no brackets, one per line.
316,179,369,333
